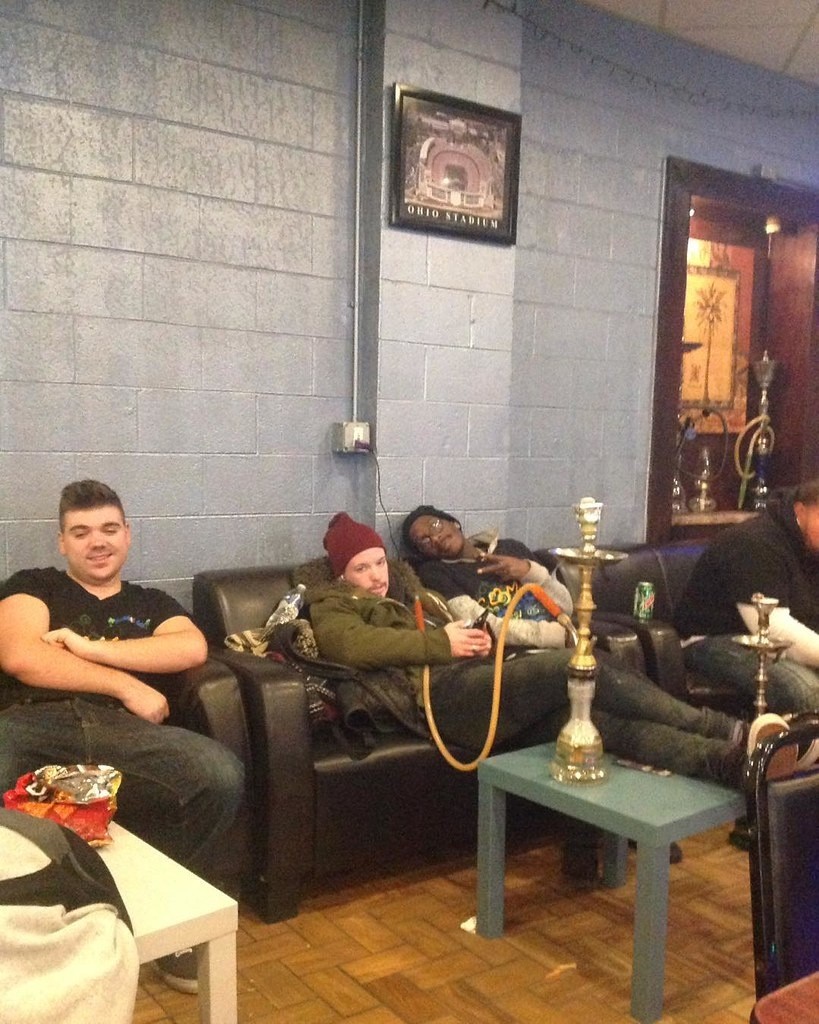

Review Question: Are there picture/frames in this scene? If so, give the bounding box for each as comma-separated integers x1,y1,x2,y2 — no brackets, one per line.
388,82,522,247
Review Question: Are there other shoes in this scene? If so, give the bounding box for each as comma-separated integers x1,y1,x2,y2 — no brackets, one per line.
629,832,684,864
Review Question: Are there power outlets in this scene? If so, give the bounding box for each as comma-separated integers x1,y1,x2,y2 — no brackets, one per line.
332,422,370,455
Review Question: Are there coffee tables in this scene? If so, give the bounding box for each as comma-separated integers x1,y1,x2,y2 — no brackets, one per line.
476,741,749,1024
92,819,239,1024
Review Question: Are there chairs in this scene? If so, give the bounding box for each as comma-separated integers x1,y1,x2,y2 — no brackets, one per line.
747,712,819,1024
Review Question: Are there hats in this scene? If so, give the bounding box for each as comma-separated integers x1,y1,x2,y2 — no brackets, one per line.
323,511,387,577
401,505,461,559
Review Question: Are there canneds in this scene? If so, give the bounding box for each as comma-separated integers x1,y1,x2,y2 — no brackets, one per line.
633,581,655,621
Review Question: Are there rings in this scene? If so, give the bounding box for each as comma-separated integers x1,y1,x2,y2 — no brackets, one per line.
473,645,476,651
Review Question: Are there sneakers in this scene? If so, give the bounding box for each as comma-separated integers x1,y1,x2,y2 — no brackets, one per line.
731,712,798,792
151,947,198,994
748,705,819,772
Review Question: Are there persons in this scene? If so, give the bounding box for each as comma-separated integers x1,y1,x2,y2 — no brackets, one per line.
308,506,819,793
678,473,819,717
0,479,246,997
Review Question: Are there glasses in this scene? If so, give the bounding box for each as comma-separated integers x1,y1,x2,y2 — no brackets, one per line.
418,519,444,555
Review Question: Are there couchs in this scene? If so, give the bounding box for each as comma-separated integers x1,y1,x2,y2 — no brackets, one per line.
143,540,777,925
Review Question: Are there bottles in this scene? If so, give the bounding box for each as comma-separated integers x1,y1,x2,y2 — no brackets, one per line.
265,583,306,626
